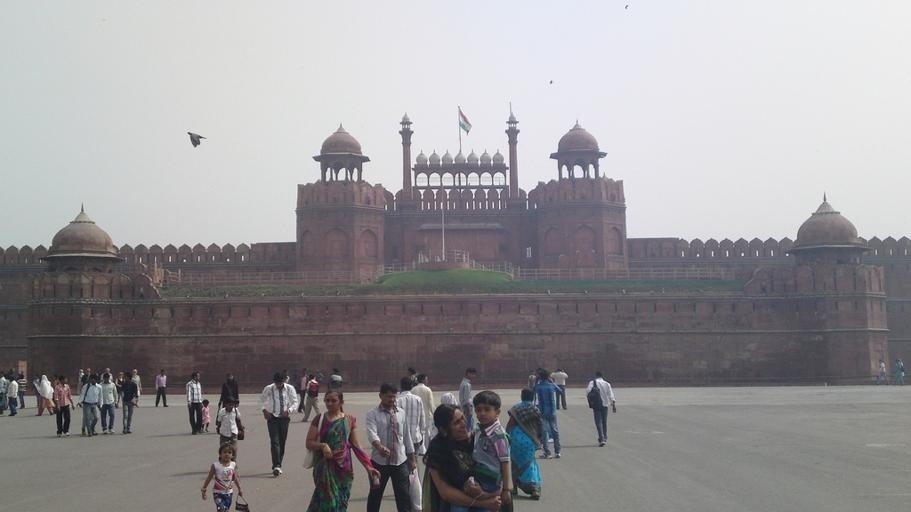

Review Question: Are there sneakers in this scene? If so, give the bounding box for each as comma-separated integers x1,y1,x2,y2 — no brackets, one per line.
56,429,131,437
273,467,282,477
539,439,606,460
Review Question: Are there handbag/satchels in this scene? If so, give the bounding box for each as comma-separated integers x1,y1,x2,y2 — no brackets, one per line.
302,450,314,469
235,496,248,512
587,387,601,409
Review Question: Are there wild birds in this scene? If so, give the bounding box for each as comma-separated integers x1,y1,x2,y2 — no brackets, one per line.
187,132,207,148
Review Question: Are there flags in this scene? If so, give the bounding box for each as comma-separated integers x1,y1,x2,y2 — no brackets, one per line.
458,107,473,133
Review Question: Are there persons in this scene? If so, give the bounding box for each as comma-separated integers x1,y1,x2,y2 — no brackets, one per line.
201,370,515,511
876,360,890,384
894,359,906,385
0,369,26,416
508,368,569,500
586,372,615,446
185,371,210,434
33,368,141,438
155,370,169,407
